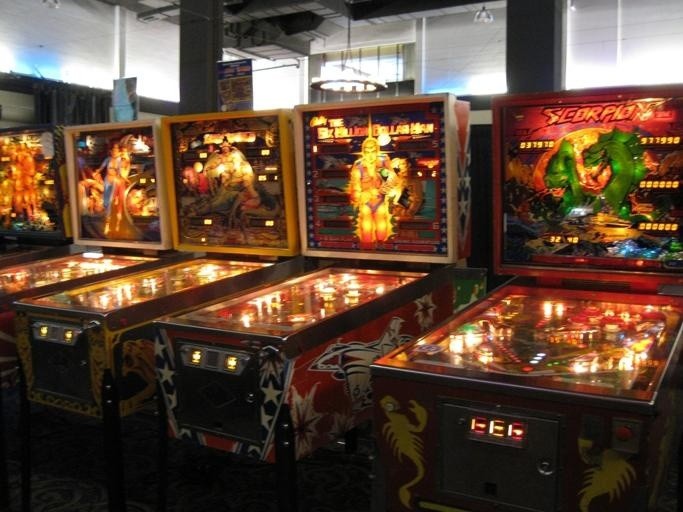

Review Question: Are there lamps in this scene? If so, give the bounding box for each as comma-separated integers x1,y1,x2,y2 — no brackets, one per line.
474,4,493,25
308,17,388,94
